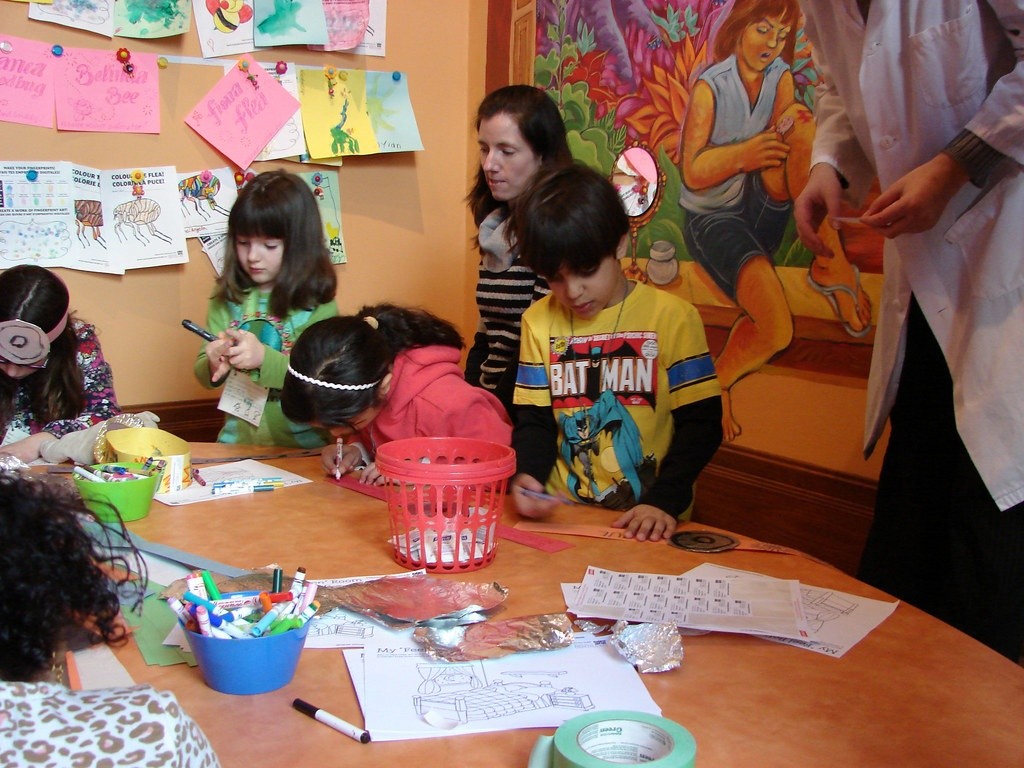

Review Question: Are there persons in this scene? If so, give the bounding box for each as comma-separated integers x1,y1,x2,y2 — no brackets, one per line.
0,468,224,768
280,304,513,493
193,170,344,449
464,85,575,417
0,264,120,464
794,0,1024,666
510,168,723,542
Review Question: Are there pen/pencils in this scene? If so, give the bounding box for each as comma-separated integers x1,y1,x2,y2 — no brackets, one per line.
518,488,576,505
212,476,285,494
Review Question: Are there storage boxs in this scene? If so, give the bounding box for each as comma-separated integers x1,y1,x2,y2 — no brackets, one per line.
105,426,193,497
72,462,162,524
178,589,309,696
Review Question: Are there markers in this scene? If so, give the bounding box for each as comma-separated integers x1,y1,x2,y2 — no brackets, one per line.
74,457,164,484
191,467,207,487
292,697,371,744
181,319,219,342
166,564,321,639
335,436,343,484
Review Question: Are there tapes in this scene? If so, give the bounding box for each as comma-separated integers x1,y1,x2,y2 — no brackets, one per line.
554,709,697,768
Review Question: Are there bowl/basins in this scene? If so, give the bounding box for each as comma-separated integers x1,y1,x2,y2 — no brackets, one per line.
74,462,161,522
178,588,313,694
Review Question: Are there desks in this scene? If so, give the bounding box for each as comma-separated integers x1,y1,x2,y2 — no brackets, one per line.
1,431,1023,768
